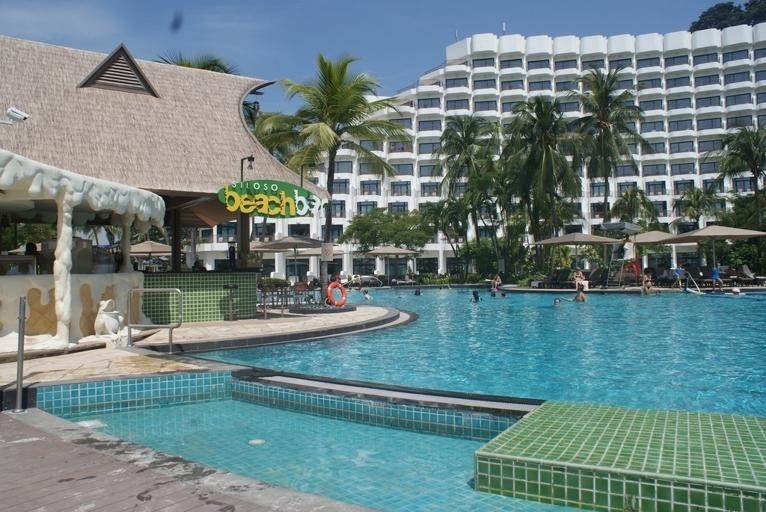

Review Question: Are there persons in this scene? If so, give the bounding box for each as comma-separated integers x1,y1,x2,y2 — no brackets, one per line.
642,269,662,295
554,267,587,306
363,289,371,301
472,290,482,302
490,273,506,297
711,263,723,294
731,286,741,296
672,263,683,289
415,287,421,295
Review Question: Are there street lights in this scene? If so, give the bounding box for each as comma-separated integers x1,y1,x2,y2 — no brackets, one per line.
239,154,255,250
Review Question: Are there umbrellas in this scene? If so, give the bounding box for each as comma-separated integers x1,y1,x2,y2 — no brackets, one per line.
366,244,420,286
251,233,339,282
529,225,766,269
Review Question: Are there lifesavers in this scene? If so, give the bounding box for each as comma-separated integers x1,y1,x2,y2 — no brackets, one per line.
624,262,641,279
327,282,347,307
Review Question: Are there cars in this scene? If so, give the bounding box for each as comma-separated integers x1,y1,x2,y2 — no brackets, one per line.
615,260,737,283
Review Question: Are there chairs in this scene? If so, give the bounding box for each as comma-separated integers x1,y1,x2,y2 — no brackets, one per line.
648,264,766,289
530,268,602,289
